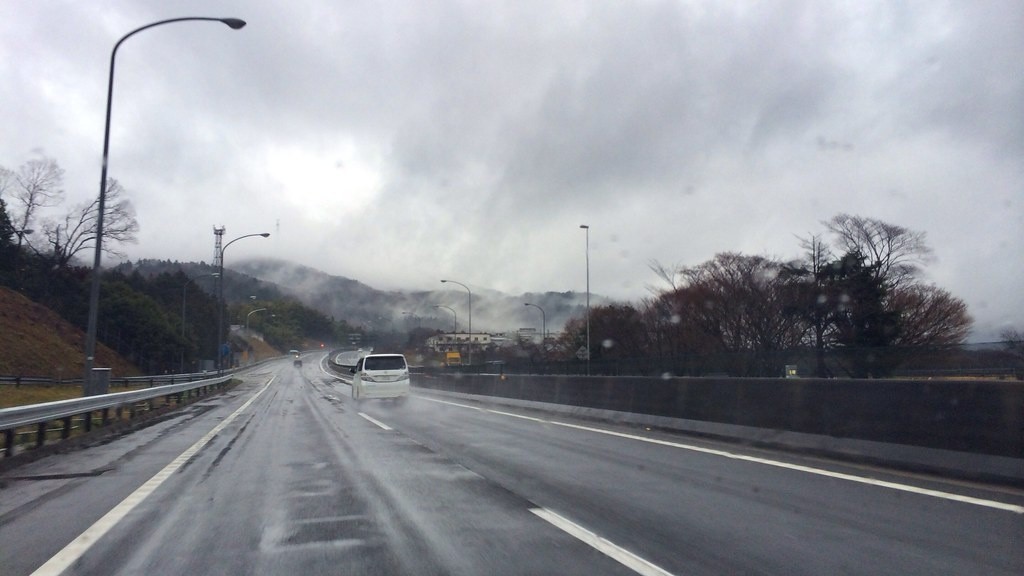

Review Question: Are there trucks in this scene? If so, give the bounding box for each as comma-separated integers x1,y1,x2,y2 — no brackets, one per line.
442,352,462,368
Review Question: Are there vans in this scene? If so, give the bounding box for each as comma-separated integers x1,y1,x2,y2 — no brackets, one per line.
349,353,410,408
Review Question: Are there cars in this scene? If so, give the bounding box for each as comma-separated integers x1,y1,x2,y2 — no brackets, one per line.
358,347,363,352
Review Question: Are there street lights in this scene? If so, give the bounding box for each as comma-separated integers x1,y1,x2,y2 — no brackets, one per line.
246,308,267,362
402,312,421,364
524,303,546,375
218,232,270,376
441,280,471,365
83,16,246,397
579,225,591,377
179,272,221,375
433,305,457,352
